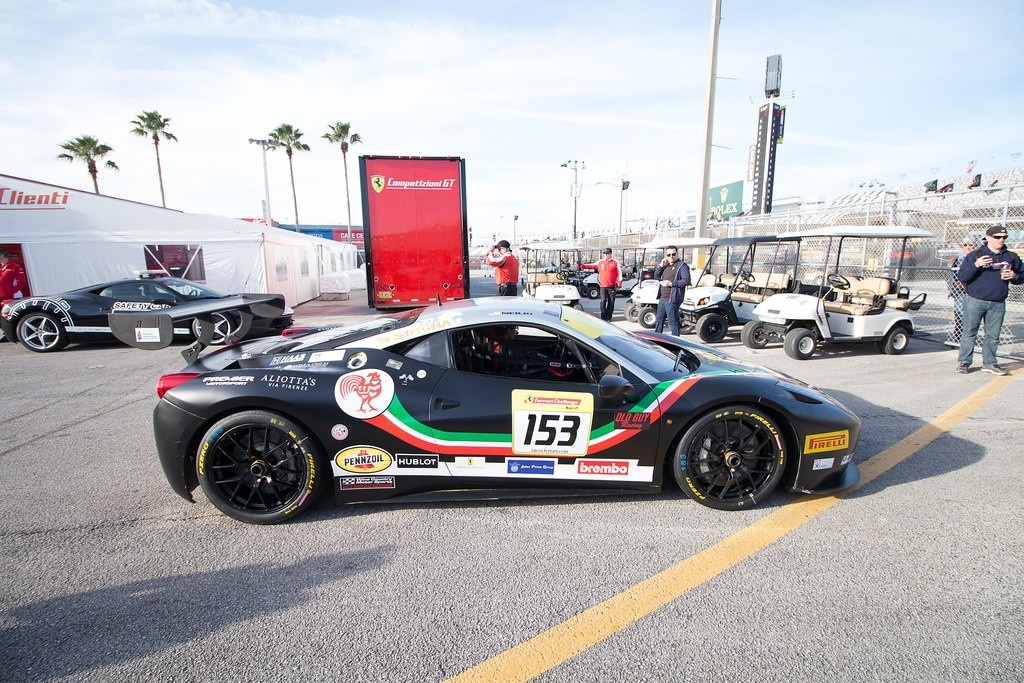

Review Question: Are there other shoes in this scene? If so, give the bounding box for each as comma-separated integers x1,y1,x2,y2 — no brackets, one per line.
981,364,1007,375
956,364,968,374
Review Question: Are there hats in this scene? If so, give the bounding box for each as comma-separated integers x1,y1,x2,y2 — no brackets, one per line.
602,248,612,253
495,240,510,248
982,226,1007,240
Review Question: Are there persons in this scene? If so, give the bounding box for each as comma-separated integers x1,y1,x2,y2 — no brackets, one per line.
577,248,622,322
480,325,575,376
654,246,690,337
949,235,976,342
486,240,519,297
955,226,1024,376
0,248,26,342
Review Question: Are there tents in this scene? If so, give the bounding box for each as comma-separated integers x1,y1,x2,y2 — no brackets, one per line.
0,174,358,315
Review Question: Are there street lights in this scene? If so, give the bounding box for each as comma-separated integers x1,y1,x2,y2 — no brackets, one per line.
560,159,584,240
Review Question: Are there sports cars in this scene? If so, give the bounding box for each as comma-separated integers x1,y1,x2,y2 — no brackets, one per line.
0,269,294,355
149,294,861,528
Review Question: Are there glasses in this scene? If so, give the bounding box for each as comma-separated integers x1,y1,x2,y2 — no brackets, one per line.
667,253,676,257
991,235,1008,239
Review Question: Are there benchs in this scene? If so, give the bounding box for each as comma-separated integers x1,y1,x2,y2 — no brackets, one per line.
528,271,564,296
730,272,791,304
824,275,892,316
691,268,736,291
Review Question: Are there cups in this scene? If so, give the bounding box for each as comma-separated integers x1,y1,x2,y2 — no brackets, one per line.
1000,264,1012,280
